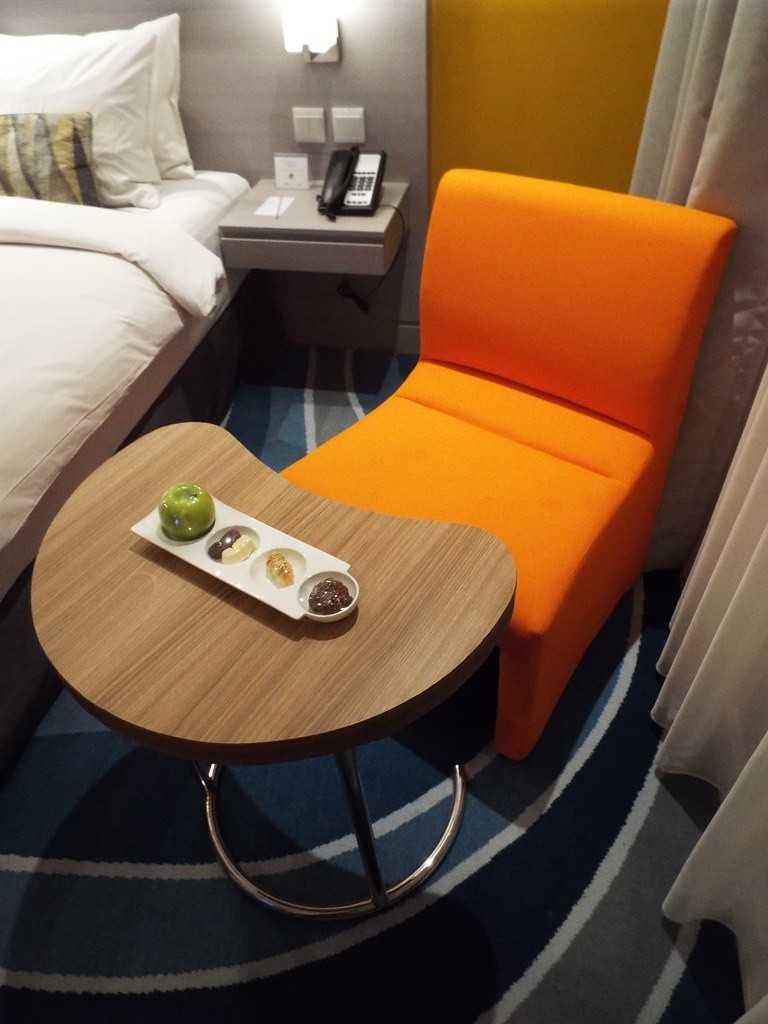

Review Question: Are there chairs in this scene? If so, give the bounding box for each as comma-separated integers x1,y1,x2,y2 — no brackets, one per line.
282,168,738,761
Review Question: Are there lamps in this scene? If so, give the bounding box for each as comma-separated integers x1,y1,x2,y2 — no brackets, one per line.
280,12,343,64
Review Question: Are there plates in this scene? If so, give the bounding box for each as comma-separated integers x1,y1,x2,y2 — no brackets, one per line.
131,493,350,620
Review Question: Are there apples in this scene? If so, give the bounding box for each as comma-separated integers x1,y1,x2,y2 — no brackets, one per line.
157,482,215,540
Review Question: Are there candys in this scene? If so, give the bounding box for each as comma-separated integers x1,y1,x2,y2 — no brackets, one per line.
208,530,255,565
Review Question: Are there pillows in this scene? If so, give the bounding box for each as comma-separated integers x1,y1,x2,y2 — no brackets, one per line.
0,12,199,181
1,112,116,207
0,38,162,214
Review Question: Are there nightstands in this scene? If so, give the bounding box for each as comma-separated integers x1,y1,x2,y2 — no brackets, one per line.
223,170,407,391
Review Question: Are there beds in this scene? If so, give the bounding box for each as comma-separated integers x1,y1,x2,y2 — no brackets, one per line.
0,171,256,604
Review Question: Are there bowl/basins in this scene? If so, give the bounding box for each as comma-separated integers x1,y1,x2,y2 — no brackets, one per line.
297,570,361,622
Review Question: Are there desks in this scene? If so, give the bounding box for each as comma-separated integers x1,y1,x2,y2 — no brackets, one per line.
30,417,516,920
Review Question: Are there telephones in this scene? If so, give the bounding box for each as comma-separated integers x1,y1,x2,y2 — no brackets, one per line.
316,144,387,221
